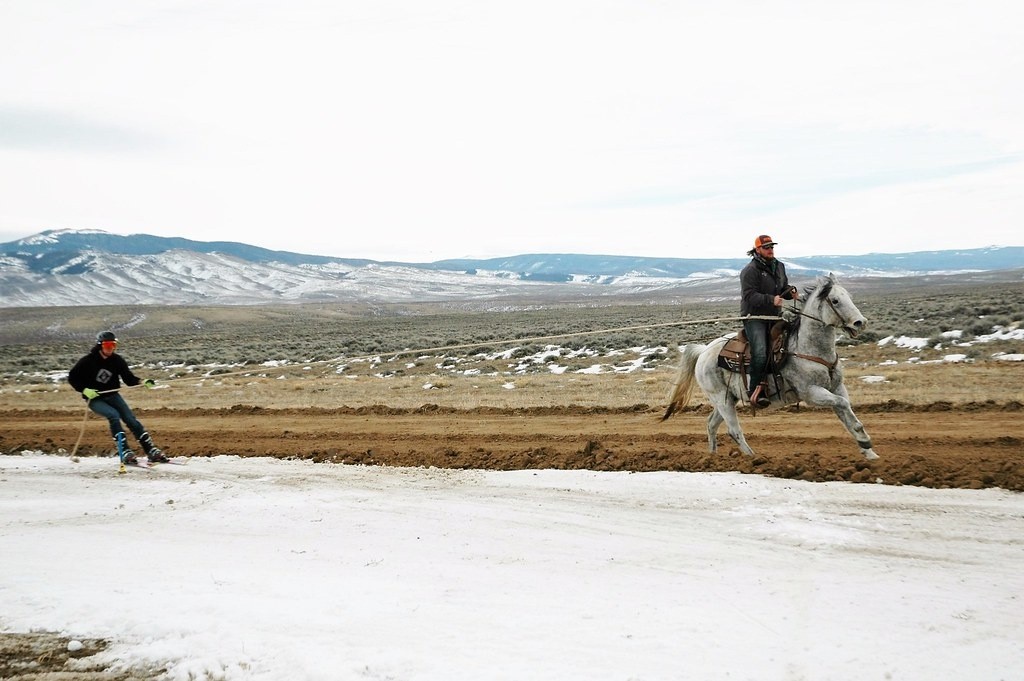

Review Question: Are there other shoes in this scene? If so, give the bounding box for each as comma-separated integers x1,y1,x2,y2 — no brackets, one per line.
747,390,770,404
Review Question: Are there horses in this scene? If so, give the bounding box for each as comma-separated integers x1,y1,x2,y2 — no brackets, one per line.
654,271,881,462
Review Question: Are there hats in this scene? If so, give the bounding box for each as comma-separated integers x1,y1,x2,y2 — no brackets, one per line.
97,331,117,346
755,235,778,248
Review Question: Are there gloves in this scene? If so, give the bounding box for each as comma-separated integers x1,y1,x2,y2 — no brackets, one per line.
83,388,100,399
142,378,157,388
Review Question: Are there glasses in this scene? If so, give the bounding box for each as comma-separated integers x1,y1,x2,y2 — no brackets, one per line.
102,341,116,349
762,244,773,249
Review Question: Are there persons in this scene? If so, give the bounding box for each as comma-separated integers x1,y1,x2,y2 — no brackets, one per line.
739,235,799,408
68,331,171,465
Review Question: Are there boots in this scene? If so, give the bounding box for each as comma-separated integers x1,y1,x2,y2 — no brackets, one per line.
112,432,135,463
136,432,166,460
